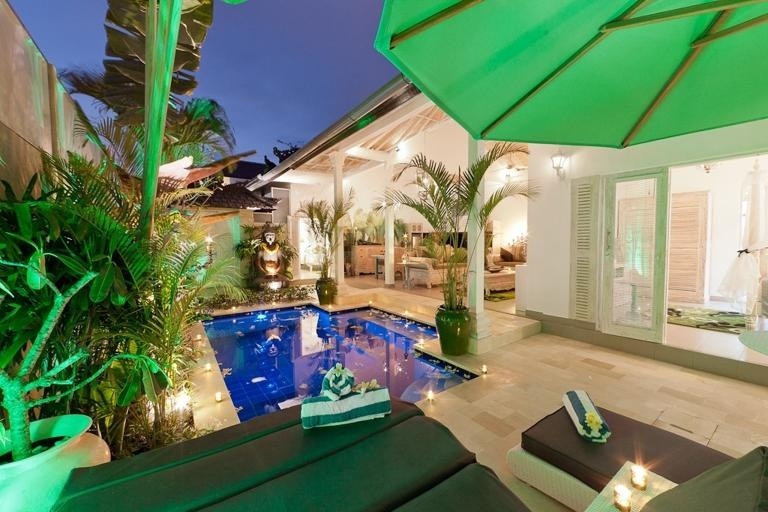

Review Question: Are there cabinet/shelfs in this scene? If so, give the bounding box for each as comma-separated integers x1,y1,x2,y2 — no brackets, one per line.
351,244,405,276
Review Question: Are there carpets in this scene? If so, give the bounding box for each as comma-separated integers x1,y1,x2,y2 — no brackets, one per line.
440,286,517,302
641,304,761,333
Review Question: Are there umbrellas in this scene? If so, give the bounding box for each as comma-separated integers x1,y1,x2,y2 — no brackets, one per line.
372,0,768,147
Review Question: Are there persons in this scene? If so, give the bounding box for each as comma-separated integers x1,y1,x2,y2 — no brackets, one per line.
253,230,290,289
256,313,289,358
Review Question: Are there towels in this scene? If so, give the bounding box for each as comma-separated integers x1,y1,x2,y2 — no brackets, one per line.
300,368,395,430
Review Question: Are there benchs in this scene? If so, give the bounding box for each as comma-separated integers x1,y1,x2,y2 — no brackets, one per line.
506,402,737,504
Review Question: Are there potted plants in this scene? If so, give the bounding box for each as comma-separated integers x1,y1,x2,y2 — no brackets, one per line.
0,200,111,512
293,186,355,304
371,139,538,356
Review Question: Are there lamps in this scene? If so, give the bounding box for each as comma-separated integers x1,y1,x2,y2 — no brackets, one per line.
548,151,568,177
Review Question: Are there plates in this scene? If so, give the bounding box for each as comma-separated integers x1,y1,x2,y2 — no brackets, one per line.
486,266,505,274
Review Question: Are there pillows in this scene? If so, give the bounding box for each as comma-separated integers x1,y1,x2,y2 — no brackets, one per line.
563,389,606,439
500,248,513,260
512,240,527,261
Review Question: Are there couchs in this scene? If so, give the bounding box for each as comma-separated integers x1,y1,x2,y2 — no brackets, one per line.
403,244,466,289
53,392,768,512
487,245,527,268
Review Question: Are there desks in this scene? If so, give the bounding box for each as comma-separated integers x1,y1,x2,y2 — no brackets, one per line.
484,269,515,295
396,260,419,288
371,254,384,279
